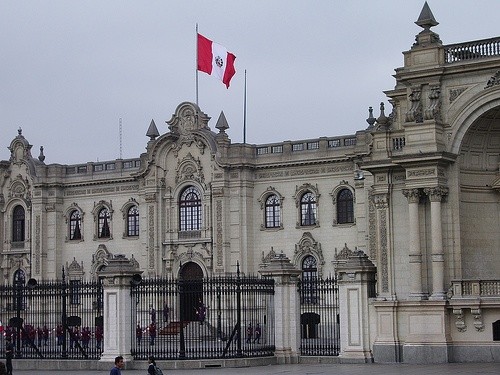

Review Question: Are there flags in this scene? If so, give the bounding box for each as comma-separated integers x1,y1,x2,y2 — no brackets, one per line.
196,33,237,89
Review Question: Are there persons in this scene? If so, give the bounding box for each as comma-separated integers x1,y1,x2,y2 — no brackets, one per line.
254,322,262,344
147,356,163,375
4,323,103,354
110,356,124,375
5,346,14,375
137,322,157,347
162,303,170,323
149,304,157,325
246,323,253,344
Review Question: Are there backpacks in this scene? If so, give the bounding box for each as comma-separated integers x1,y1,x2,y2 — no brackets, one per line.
154,366,163,375
